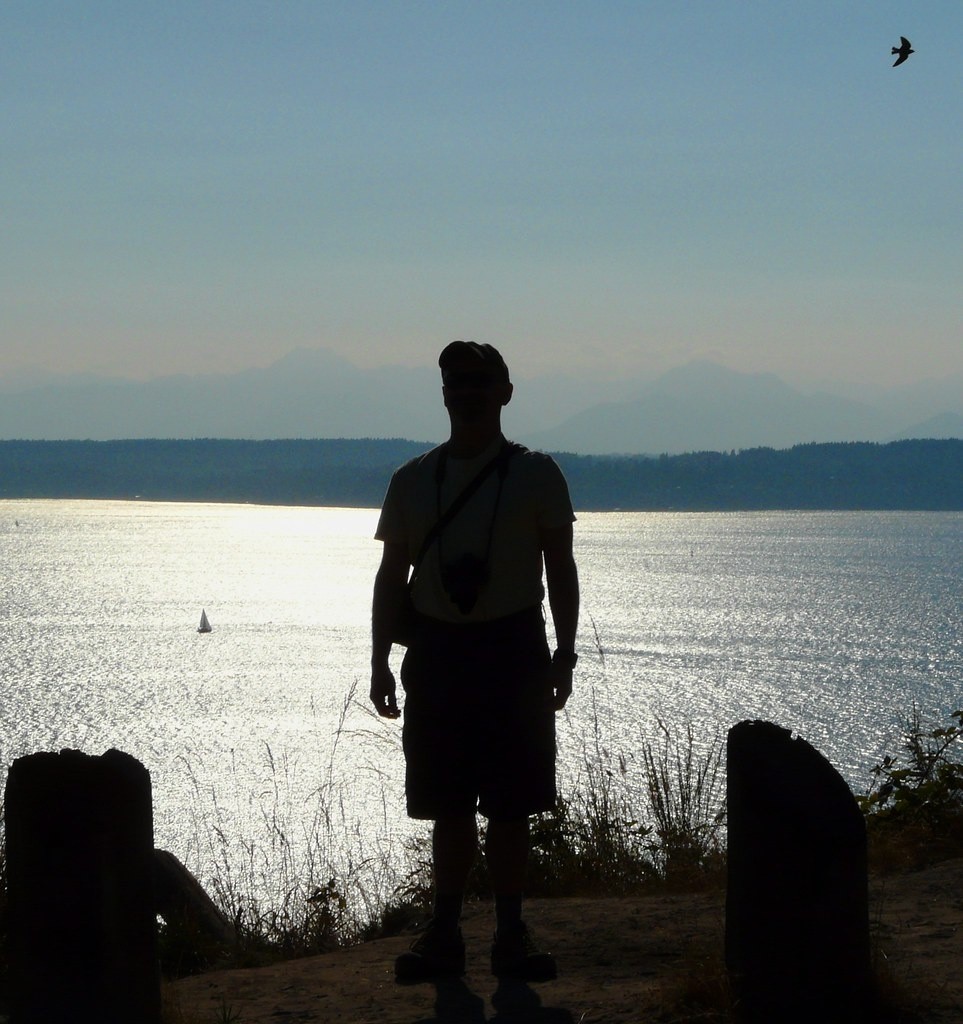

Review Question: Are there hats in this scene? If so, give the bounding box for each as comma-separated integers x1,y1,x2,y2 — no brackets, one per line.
439,341,508,382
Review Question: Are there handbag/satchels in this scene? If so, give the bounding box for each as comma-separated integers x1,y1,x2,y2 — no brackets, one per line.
393,586,415,645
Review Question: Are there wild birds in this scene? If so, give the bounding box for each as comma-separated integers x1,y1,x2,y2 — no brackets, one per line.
891,35,916,67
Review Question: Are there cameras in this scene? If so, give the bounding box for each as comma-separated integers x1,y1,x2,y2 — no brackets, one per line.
440,551,492,616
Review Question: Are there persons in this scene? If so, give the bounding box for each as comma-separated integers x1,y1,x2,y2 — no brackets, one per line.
368,341,580,986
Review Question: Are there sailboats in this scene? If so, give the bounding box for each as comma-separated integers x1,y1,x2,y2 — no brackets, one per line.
197,608,212,634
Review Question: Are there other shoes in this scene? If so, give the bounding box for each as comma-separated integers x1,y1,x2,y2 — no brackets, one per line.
396,927,466,985
492,921,558,979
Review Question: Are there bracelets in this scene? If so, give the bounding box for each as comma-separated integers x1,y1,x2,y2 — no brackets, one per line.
554,648,578,665
